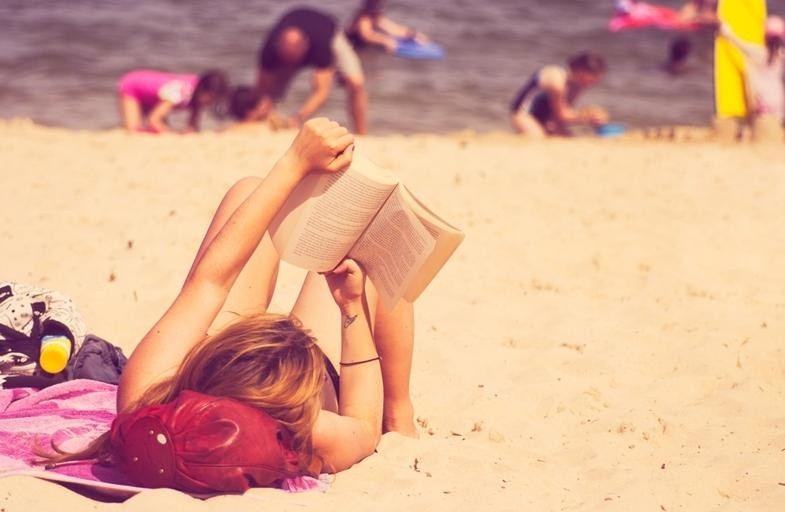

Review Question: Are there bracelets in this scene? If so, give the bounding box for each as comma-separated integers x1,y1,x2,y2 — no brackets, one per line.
340,357,382,367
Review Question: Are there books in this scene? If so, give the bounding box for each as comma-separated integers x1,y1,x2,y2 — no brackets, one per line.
268,159,464,314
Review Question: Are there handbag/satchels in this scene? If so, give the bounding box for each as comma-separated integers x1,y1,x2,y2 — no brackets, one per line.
1,281,88,376
107,391,313,494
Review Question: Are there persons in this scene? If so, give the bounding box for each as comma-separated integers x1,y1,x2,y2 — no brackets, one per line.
116,70,226,135
33,118,418,475
